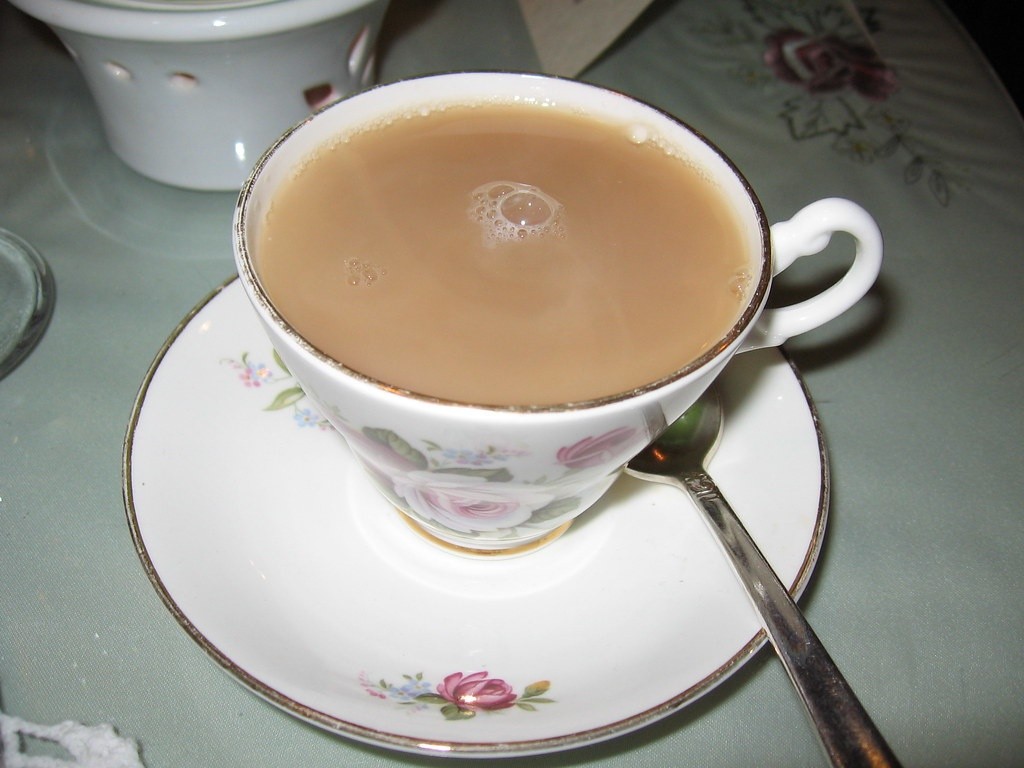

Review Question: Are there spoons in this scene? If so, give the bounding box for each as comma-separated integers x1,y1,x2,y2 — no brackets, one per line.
622,380,902,768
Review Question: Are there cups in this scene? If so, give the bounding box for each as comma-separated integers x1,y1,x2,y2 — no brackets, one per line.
231,69,886,561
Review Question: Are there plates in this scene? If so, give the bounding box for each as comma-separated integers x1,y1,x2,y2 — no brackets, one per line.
123,268,833,758
